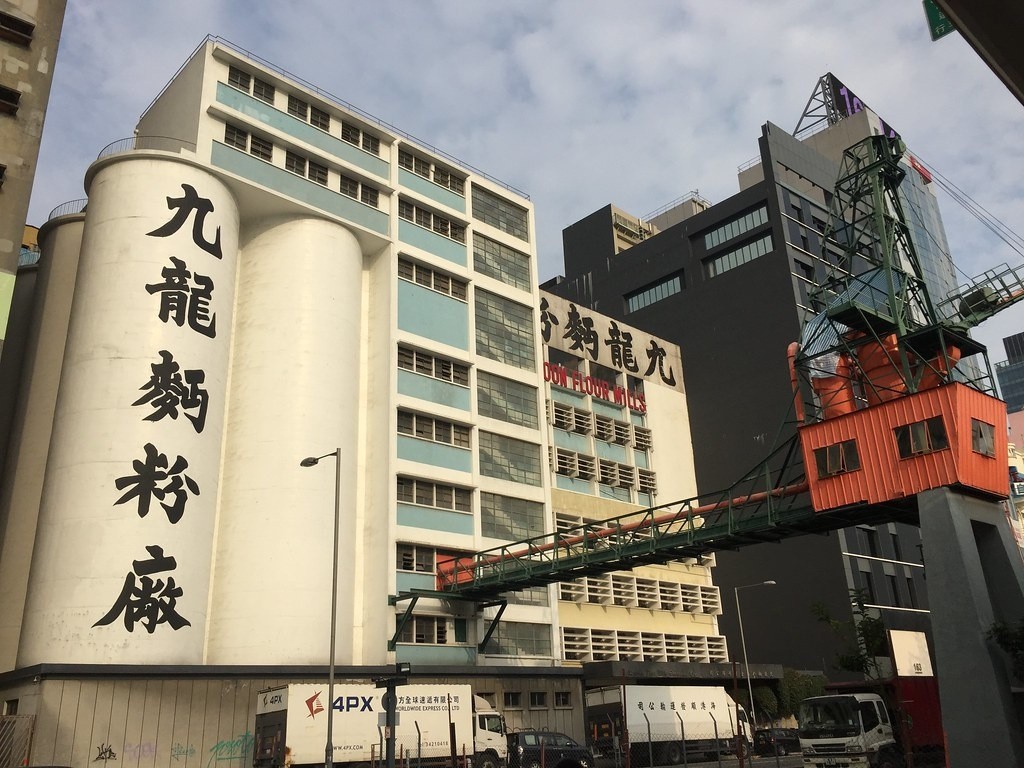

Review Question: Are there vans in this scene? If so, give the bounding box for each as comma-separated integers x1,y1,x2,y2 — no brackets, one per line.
507,731,595,768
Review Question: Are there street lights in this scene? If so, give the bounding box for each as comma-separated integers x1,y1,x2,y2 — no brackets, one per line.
300,447,342,768
734,581,776,727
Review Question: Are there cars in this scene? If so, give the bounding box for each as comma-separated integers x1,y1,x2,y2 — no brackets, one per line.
755,727,799,758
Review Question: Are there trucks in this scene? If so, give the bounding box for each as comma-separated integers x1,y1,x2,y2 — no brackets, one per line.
255,682,509,768
797,676,943,768
586,684,756,765
